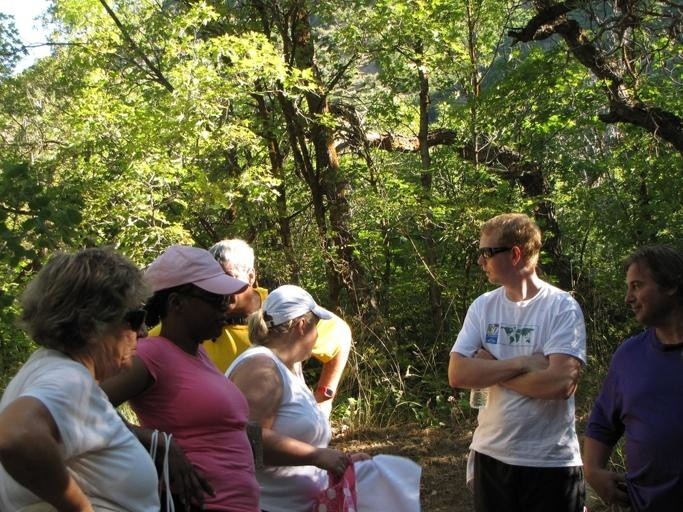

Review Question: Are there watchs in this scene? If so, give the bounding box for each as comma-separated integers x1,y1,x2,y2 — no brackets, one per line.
317,385,334,399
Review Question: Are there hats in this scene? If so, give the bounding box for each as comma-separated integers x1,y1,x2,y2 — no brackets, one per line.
262,285,332,328
145,246,248,296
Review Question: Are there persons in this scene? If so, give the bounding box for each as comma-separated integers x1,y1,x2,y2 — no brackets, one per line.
224,284,370,512
581,243,683,512
0,250,162,511
96,244,260,512
142,238,352,418
448,211,587,512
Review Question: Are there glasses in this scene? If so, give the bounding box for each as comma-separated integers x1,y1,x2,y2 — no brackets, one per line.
475,246,509,259
124,309,146,333
186,289,232,307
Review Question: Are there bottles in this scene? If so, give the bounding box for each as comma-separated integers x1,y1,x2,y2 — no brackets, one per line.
467,387,490,408
